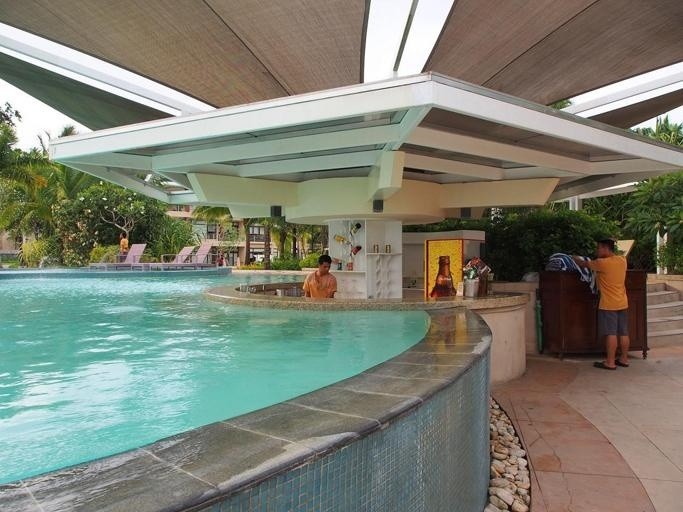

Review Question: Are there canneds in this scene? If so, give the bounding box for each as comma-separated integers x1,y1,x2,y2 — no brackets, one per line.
386,245,391,253
374,245,379,253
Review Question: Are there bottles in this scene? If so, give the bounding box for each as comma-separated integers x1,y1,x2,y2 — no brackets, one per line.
330,222,362,270
429,256,457,299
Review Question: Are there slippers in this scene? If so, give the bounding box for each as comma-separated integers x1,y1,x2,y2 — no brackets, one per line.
615,359,630,367
593,361,616,370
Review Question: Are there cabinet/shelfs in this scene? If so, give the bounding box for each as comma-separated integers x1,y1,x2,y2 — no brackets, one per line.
328,220,402,299
540,267,649,359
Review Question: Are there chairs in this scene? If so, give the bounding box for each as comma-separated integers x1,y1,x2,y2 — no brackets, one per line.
89,243,216,271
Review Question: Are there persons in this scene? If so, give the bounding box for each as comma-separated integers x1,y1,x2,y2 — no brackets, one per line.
117,230,128,261
300,255,338,299
571,238,631,371
